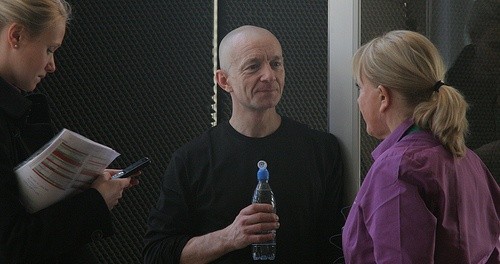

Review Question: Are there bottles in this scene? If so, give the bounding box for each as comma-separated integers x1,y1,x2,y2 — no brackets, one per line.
251,160,276,260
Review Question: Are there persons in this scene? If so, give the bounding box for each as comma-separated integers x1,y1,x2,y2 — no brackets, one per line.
0,0,141,264
444,1,500,186
340,29,500,264
142,25,348,264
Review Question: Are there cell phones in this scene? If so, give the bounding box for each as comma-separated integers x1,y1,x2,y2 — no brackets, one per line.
111,157,151,178
